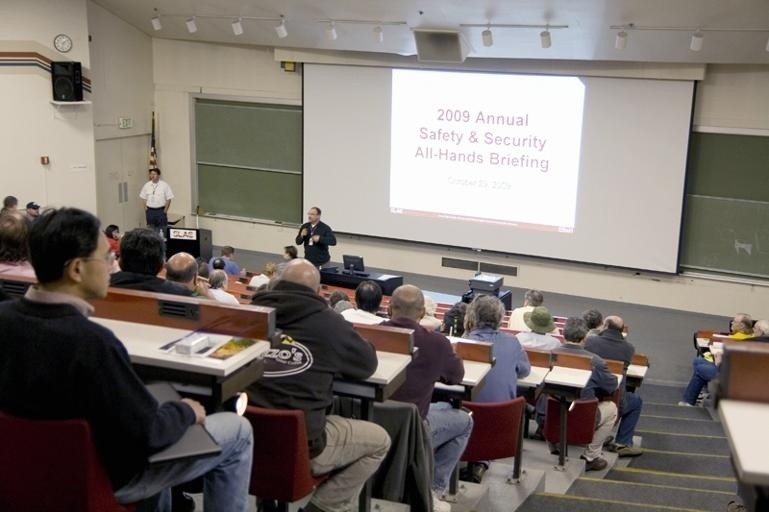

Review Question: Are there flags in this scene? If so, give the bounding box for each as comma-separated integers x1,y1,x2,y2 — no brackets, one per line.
146,118,160,182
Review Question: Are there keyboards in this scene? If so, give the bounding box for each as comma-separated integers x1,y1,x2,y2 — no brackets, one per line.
343,270,369,277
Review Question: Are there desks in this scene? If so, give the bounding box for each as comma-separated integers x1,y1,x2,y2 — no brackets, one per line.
694,333,721,364
716,400,768,486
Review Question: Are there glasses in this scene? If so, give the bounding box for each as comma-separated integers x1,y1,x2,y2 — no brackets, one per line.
64,249,115,268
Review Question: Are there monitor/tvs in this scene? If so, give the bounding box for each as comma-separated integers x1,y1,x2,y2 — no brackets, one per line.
343,254,364,275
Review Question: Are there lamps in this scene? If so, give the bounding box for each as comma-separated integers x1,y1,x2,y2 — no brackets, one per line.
459,24,570,49
151,14,288,41
318,18,407,45
609,25,769,53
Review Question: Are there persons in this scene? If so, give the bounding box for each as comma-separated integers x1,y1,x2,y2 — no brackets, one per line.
676,312,768,414
137,169,173,241
3,192,650,512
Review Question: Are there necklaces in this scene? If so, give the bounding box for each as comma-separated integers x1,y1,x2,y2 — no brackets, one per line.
148,182,162,195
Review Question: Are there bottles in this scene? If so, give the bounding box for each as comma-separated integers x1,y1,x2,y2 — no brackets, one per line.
242,268,246,278
160,229,163,238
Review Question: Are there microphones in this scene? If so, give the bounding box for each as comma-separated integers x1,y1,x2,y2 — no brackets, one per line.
196,205,200,229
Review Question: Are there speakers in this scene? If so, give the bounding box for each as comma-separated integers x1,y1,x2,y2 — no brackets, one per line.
51,61,83,102
413,28,471,63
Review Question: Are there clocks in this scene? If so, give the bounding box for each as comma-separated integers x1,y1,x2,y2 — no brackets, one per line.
53,33,72,53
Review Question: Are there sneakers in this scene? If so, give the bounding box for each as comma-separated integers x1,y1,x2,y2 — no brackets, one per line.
581,455,607,471
431,490,451,511
602,434,643,458
677,401,697,408
728,501,747,512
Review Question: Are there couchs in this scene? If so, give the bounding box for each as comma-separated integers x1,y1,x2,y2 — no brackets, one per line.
704,339,768,411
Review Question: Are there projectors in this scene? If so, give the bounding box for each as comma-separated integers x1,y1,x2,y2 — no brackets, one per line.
469,271,504,291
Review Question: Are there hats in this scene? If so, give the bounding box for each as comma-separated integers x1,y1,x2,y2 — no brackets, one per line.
26,202,39,209
523,306,556,333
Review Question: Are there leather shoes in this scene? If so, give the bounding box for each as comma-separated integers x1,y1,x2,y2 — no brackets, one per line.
459,463,485,483
